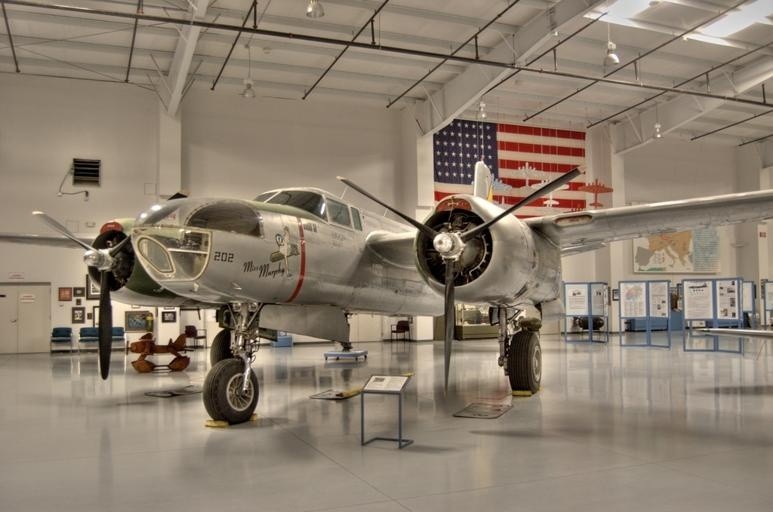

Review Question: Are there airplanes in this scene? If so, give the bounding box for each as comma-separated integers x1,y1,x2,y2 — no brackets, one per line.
32,155,773,426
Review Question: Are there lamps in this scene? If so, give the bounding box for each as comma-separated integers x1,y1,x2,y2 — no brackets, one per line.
306,0,324,18
603,18,620,67
652,102,663,139
237,46,256,99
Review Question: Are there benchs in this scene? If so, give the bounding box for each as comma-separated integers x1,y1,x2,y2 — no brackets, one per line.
78,327,126,356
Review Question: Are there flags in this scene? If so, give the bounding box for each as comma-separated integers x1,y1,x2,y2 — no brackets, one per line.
434,119,586,219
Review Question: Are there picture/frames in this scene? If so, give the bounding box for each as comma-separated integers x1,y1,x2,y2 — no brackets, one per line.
125,311,149,332
74,288,85,297
58,287,73,301
86,274,101,299
72,307,85,324
162,311,176,323
93,306,100,328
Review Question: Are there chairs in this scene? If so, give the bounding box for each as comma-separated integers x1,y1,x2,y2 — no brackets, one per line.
391,320,412,345
185,325,207,348
50,328,75,355
